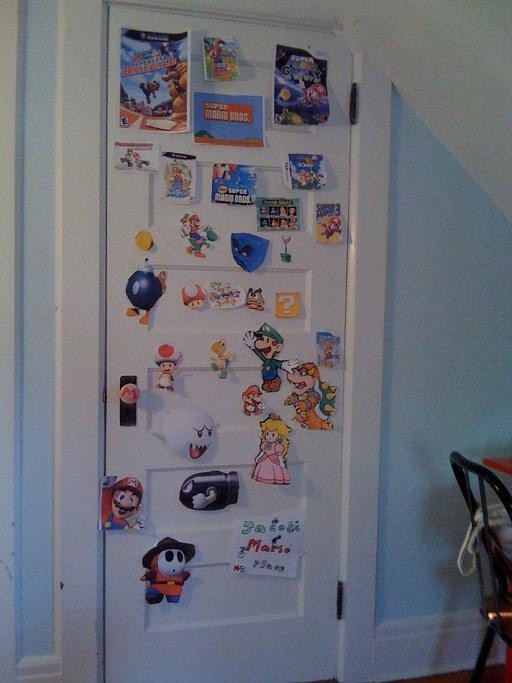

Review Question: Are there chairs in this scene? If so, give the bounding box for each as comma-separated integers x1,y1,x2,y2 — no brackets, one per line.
446,449,512,682
